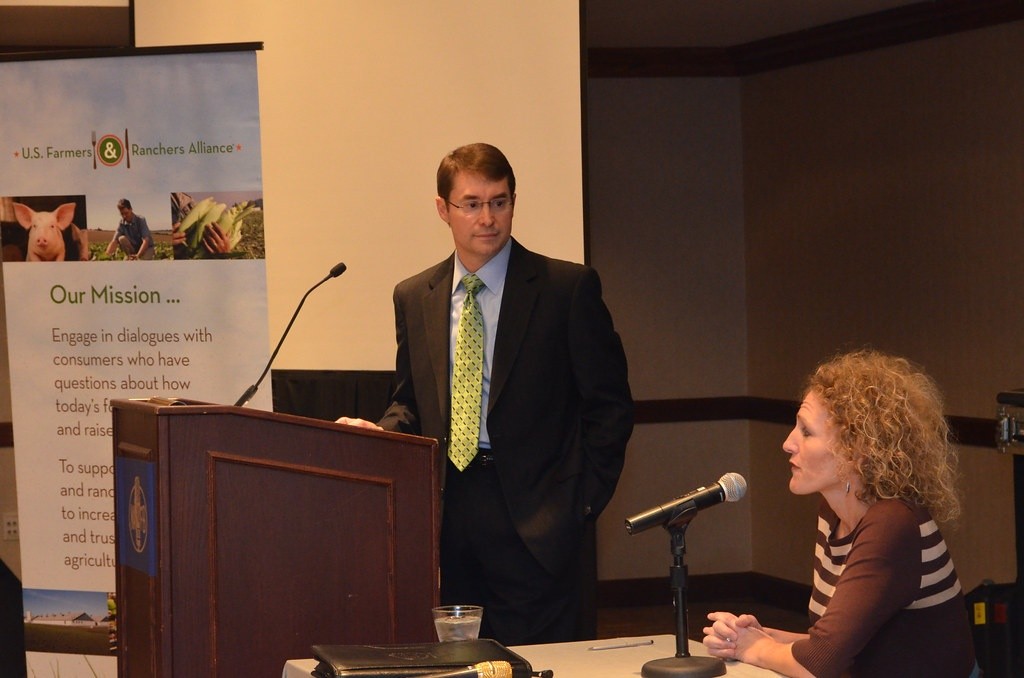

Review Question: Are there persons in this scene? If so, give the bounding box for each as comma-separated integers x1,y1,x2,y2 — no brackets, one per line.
703,348,985,678
335,142,638,648
171,192,231,260
108,200,156,260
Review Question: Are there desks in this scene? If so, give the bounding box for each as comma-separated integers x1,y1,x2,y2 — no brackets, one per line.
281,635,789,678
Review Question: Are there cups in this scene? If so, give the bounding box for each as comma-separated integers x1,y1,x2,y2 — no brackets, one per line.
431,604,484,643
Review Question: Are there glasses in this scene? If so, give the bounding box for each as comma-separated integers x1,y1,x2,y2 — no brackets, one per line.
444,195,514,213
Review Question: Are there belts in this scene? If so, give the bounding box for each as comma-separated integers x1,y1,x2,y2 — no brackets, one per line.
469,452,495,467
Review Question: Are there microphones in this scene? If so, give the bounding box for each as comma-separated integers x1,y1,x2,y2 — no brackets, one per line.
624,472,746,535
233,262,346,407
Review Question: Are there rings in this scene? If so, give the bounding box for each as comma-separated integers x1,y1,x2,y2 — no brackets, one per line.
727,636,730,642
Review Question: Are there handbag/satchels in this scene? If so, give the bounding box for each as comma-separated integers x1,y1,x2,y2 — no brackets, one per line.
310,637,534,678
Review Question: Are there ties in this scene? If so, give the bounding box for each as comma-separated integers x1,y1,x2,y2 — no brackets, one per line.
447,273,487,472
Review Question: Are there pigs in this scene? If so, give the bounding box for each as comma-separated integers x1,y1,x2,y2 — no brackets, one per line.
11,203,76,262
